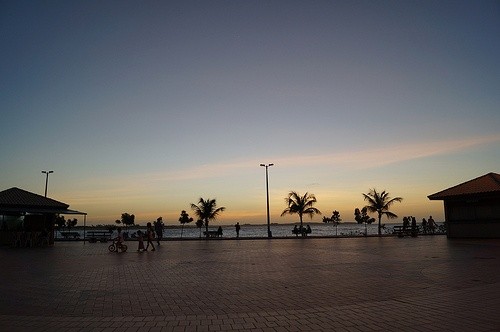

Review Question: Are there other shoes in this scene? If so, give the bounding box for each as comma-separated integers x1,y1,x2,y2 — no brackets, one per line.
114,251,118,252
136,249,139,251
143,249,147,251
151,248,155,251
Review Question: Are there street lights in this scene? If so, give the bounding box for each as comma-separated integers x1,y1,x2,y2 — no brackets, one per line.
41,170,53,198
260,164,274,236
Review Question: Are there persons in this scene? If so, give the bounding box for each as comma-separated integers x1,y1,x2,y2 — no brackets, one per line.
136,220,163,252
403,217,409,235
420,218,428,234
218,226,223,237
294,224,312,238
235,222,241,237
411,217,417,235
427,216,438,232
113,227,123,252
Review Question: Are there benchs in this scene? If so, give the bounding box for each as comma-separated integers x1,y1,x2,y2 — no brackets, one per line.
61,231,80,239
87,231,111,240
203,230,223,238
292,230,312,237
394,226,423,239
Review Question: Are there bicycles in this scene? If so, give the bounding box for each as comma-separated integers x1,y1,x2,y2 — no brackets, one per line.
108,238,128,252
